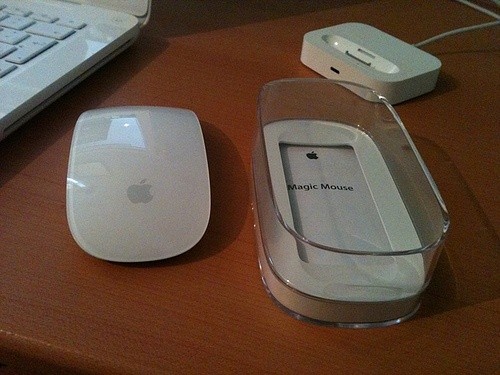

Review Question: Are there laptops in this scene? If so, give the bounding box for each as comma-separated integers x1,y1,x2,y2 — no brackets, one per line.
0,0,152,141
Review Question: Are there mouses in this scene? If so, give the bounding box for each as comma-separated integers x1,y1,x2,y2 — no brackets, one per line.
66,106,211,262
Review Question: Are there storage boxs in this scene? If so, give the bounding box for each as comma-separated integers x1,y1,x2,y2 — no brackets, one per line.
248,78,451,329
